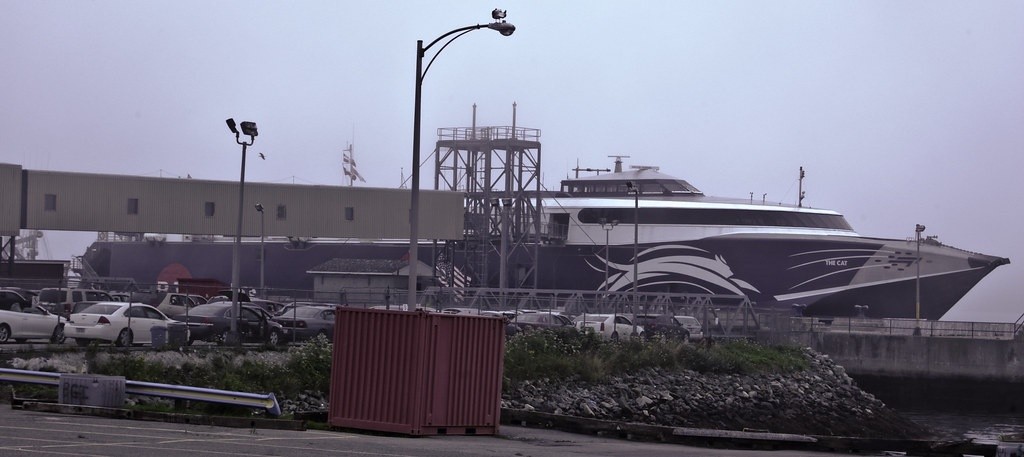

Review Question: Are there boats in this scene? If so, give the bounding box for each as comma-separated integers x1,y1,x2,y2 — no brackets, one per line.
70,101,1010,321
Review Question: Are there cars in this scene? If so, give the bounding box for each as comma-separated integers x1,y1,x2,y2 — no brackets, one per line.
63,301,190,348
368,305,705,343
176,301,288,350
0,304,71,345
0,281,343,320
274,308,353,342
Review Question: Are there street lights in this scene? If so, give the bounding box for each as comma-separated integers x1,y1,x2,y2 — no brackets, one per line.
598,217,618,299
226,118,259,332
255,203,265,288
626,180,644,343
407,7,517,311
